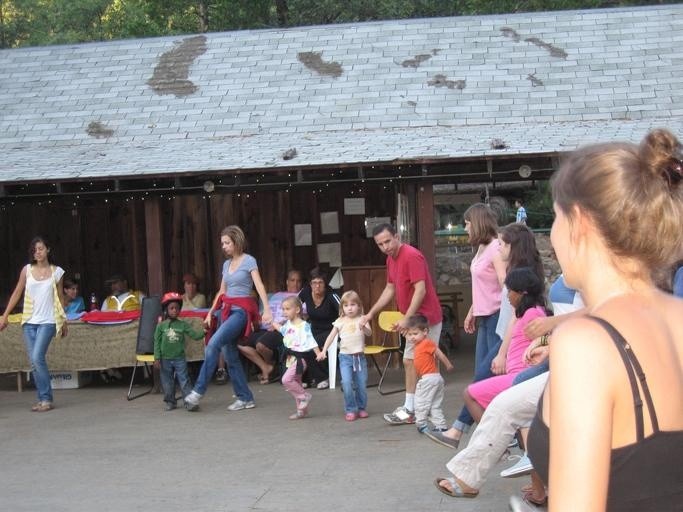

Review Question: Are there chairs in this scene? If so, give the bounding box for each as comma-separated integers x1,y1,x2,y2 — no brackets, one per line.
126,294,190,403
361,309,407,402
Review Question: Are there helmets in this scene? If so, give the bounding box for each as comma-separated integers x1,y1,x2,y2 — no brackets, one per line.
161,292,183,312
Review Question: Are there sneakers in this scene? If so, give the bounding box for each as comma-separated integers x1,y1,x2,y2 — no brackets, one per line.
384,406,548,506
165,369,368,421
31,401,54,412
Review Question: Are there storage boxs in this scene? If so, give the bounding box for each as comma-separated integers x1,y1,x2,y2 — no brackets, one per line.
44,368,92,390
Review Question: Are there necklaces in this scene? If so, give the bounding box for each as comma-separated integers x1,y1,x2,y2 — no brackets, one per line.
39,264,49,279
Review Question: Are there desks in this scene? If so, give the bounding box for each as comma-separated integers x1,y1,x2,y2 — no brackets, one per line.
1,308,208,392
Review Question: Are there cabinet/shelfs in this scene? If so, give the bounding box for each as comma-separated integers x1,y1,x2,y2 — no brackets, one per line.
338,264,400,373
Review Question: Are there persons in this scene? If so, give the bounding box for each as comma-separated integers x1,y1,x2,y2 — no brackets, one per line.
389,313,454,434
183,224,273,410
211,267,343,390
359,223,443,425
180,273,205,307
422,130,682,511
62,276,86,314
95,274,152,385
153,292,209,411
260,297,326,419
315,288,372,421
0,237,68,412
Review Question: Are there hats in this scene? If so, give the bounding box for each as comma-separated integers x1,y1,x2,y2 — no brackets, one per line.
105,275,125,283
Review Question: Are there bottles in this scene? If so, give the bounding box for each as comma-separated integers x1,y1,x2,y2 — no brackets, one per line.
88,292,98,312
